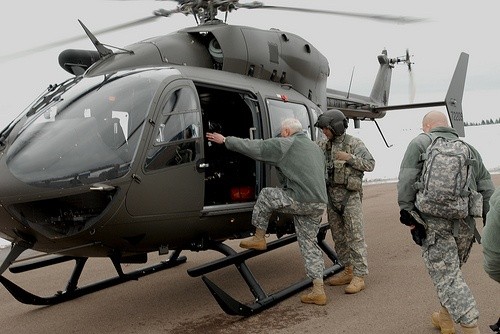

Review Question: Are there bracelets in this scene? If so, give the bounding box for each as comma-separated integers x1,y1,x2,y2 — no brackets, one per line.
222,137,226,142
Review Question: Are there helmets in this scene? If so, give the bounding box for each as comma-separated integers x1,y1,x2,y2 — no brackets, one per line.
314,109,348,136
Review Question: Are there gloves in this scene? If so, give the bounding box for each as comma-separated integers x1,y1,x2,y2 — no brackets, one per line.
399,208,428,240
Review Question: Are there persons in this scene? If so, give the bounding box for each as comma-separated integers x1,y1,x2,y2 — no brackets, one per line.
314,109,375,294
480,186,500,334
206,119,329,306
396,110,495,334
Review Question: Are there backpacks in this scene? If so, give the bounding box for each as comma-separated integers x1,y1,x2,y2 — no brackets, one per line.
415,132,475,220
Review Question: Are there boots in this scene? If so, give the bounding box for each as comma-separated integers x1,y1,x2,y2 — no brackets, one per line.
328,266,354,285
345,275,365,293
239,228,270,250
301,279,326,305
460,322,480,334
432,306,455,334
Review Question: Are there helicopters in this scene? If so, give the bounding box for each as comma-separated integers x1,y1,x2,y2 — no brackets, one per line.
0,1,469,322
